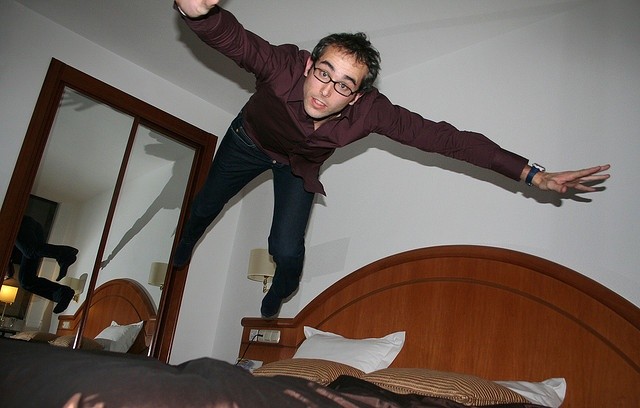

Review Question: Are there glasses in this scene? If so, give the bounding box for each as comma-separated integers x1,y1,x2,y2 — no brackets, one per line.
313,62,357,97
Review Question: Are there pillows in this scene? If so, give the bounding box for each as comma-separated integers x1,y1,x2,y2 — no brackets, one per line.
9,330,58,342
49,336,103,351
93,321,144,354
293,326,406,374
359,367,531,408
490,378,567,407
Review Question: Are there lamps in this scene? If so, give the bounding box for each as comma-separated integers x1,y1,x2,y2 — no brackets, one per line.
148,261,169,288
60,275,81,302
0,283,19,318
245,248,276,291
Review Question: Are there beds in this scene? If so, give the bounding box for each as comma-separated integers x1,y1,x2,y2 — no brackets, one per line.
2,245,640,408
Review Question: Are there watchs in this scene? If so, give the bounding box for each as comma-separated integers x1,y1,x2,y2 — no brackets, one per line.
525,162,545,187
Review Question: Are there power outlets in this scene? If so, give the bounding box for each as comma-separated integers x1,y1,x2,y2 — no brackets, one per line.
258,330,280,344
250,329,257,340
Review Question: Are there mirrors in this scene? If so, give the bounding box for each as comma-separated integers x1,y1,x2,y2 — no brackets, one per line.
0,57,137,348
252,359,365,386
74,105,217,357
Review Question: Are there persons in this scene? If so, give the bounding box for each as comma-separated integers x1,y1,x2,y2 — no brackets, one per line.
169,0,611,321
5,215,80,314
